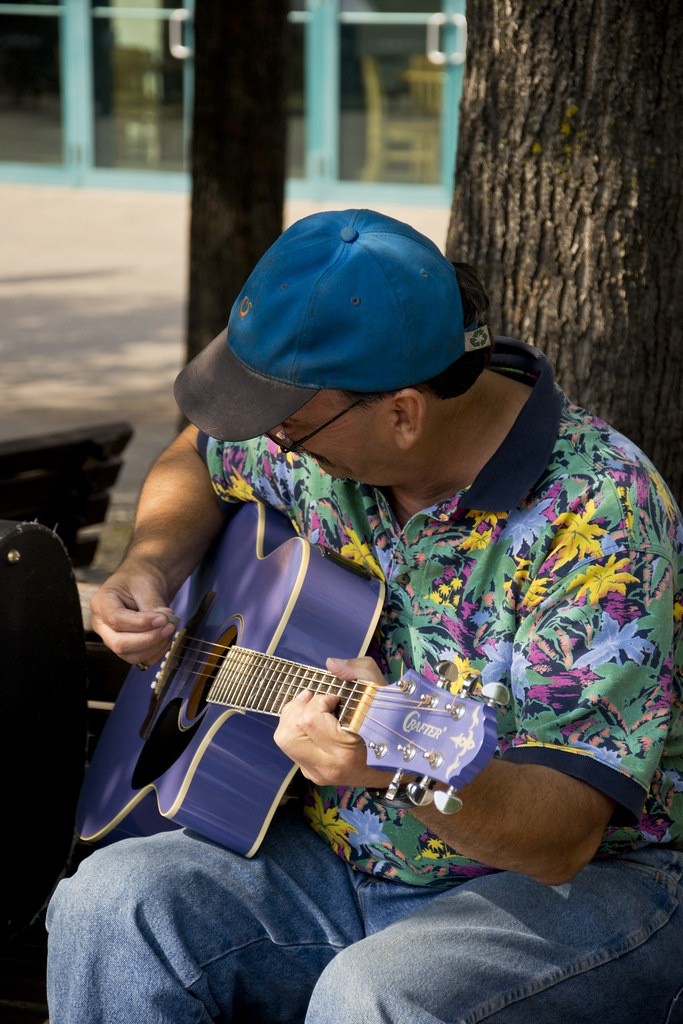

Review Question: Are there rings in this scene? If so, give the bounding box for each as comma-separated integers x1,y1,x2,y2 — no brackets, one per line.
136,663,149,672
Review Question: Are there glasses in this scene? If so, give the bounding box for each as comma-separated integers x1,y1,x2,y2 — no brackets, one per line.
264,399,366,453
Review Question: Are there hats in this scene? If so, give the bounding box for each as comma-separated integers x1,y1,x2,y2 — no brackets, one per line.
174,208,490,442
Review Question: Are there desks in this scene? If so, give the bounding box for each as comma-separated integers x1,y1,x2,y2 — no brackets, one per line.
404,56,442,114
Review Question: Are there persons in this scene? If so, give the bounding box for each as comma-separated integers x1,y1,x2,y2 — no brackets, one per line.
43,209,683,1024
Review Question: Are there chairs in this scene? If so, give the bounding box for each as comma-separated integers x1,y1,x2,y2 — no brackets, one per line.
361,54,441,184
0,421,133,569
114,43,183,170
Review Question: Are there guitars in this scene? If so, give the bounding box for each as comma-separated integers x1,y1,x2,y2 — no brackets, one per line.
77,497,500,861
